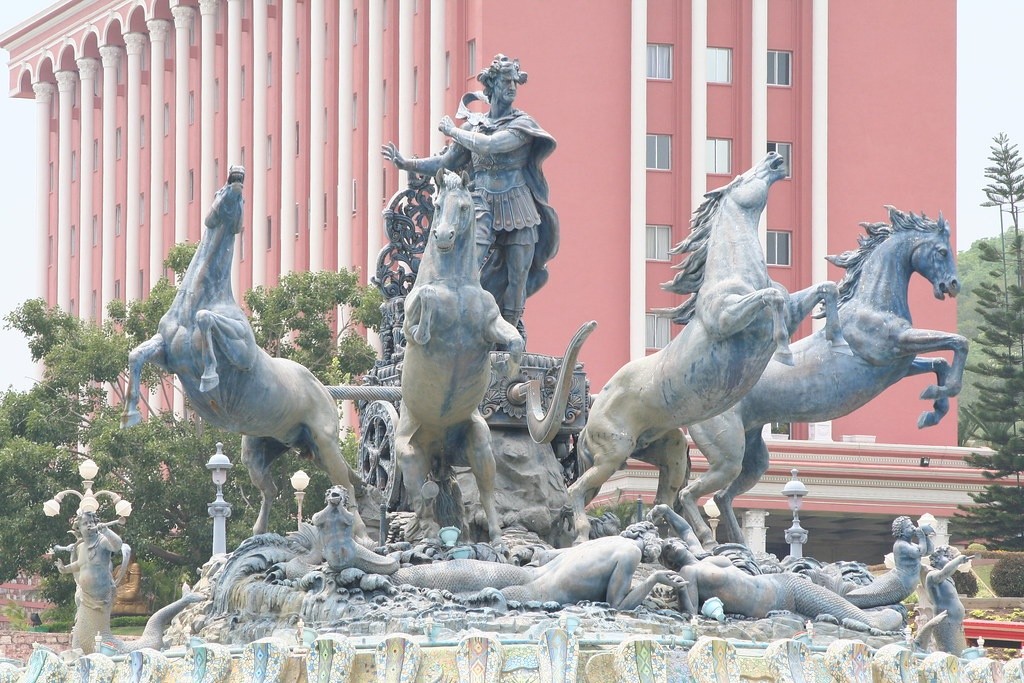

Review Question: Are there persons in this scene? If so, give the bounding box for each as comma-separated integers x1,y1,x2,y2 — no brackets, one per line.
55,483,974,658
379,53,560,331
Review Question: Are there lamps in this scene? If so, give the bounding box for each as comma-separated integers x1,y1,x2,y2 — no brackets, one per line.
882,512,972,574
921,456,930,466
291,469,311,499
704,494,722,541
43,459,134,517
701,597,728,622
438,525,461,548
781,469,810,533
204,441,235,507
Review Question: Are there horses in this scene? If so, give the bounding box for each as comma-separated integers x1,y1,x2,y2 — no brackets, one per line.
118,148,970,554
